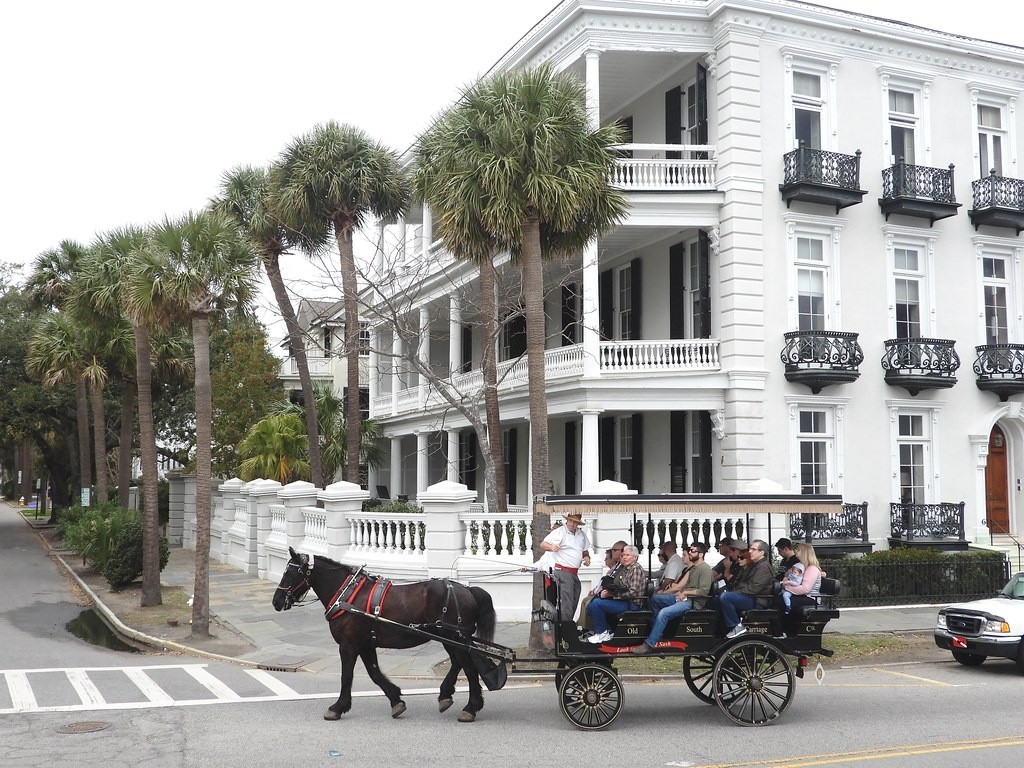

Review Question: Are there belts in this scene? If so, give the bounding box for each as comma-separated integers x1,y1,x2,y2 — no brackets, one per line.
555,563,579,574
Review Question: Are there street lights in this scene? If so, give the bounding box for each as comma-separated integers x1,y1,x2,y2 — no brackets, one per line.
35,478,42,520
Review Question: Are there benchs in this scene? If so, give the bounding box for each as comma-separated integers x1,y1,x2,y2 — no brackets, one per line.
586,572,845,635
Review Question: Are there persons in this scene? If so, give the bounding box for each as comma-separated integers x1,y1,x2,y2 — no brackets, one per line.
540,514,592,622
719,539,774,638
631,542,714,656
519,522,564,607
718,541,747,596
777,562,804,612
771,542,824,639
656,540,686,592
733,548,752,590
577,541,628,642
658,547,695,596
774,538,800,581
575,548,617,629
644,549,668,582
587,544,646,643
711,536,734,597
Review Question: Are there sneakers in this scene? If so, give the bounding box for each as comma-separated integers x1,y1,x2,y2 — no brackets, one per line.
725,626,747,638
578,632,594,641
588,630,610,644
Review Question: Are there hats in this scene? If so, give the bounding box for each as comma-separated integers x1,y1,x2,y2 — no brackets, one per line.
546,524,562,531
563,513,585,525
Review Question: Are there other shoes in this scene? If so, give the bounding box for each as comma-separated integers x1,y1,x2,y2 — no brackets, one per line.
632,640,653,654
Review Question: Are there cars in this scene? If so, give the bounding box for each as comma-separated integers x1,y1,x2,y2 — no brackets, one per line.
934,571,1024,673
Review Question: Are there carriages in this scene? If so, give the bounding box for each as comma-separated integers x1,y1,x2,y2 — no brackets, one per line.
273,493,846,733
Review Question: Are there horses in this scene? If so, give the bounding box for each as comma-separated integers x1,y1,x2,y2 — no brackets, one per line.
271,546,497,722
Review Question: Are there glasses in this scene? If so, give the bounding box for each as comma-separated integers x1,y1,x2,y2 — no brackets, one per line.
749,548,759,551
611,549,623,551
729,548,740,552
689,549,702,552
737,555,745,560
